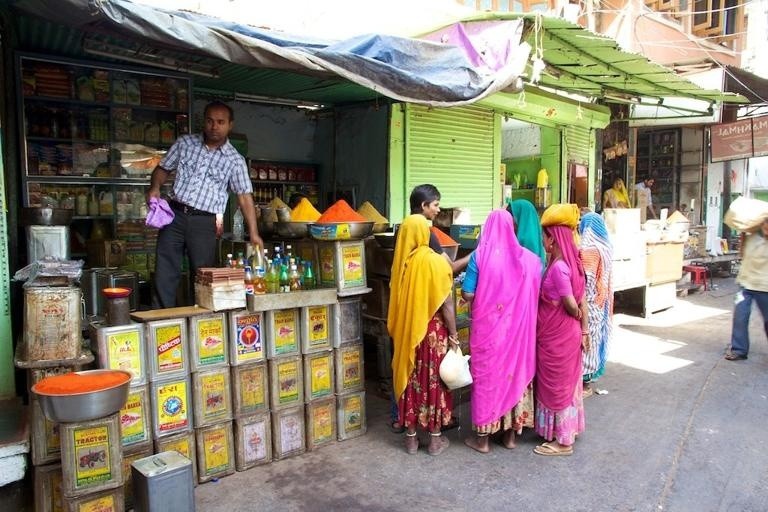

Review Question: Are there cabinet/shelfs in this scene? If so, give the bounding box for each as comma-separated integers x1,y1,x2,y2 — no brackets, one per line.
15,51,192,308
247,158,324,216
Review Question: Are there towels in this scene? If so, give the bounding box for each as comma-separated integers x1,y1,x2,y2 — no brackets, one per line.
142,194,175,229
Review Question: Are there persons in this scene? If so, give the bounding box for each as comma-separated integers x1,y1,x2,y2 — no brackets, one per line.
724,216,768,362
579,212,615,400
145,100,265,310
634,173,660,225
506,198,545,276
603,176,633,210
387,213,460,457
387,183,471,434
533,202,590,457
460,209,543,453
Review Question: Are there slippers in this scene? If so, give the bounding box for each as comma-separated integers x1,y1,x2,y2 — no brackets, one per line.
533,443,572,456
576,432,580,438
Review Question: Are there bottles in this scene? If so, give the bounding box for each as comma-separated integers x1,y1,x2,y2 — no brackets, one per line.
300,261,305,289
286,245,292,258
248,251,255,265
279,265,291,292
237,253,244,267
234,205,244,241
252,181,282,203
77,190,88,215
273,246,282,259
224,254,234,267
290,266,301,290
304,262,314,289
263,249,272,260
252,245,265,269
295,257,301,264
250,165,296,180
284,185,300,203
25,104,71,138
89,189,99,216
265,263,278,293
246,268,254,294
254,275,265,294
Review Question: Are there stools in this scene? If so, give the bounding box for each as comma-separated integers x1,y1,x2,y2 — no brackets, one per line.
682,266,707,291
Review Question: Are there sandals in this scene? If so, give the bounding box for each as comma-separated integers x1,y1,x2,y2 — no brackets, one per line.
387,416,404,434
442,416,461,429
582,388,593,401
427,433,449,455
404,434,420,454
723,353,747,361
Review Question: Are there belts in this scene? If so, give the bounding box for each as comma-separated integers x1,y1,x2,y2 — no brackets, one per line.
170,199,215,217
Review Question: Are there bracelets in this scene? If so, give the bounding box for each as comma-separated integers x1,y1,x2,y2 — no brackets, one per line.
576,309,582,320
582,331,588,336
448,332,460,345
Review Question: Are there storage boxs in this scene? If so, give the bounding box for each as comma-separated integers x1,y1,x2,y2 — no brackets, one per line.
194,281,246,312
648,242,683,286
649,280,677,314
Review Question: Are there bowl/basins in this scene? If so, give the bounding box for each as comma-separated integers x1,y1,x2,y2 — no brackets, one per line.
23,205,76,227
30,367,133,424
306,220,377,243
272,218,314,239
371,221,392,233
